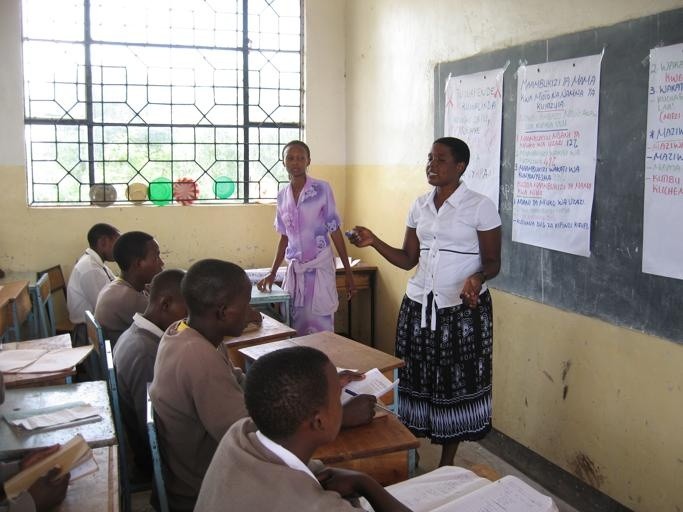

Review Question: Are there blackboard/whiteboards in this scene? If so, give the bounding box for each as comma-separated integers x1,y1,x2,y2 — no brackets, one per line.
433,8,682,346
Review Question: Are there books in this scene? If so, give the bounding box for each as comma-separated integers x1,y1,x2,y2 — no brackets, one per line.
339,365,399,401
0,343,96,373
2,395,104,434
355,463,560,511
2,432,101,504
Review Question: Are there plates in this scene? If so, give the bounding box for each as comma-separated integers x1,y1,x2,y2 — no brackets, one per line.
174,177,200,205
213,176,235,198
88,182,118,206
147,175,172,206
125,182,148,204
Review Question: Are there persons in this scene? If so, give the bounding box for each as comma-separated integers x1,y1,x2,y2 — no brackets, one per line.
94,230,164,349
1,442,71,512
112,269,187,475
65,222,121,374
149,258,252,510
256,140,358,337
191,346,412,512
346,136,502,474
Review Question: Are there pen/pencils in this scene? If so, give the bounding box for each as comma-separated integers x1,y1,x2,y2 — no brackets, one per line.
345,389,403,419
